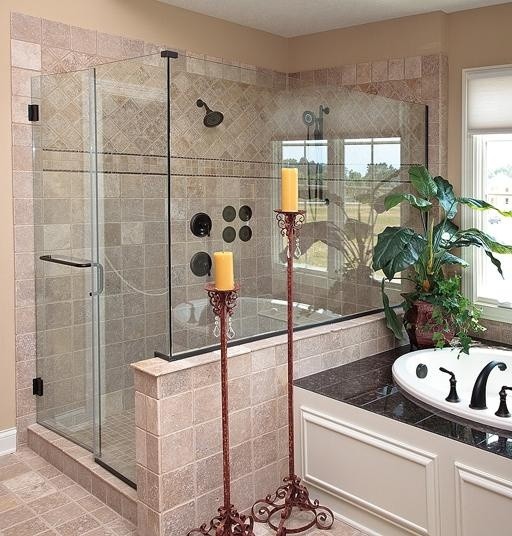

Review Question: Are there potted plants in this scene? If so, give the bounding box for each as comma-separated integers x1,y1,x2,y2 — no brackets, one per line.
371,165,512,350
278,170,409,318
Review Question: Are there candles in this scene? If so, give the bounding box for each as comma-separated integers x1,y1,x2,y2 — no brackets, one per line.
214,250,234,290
282,167,298,212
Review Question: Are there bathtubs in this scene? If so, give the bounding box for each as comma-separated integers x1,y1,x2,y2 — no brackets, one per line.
170,294,342,345
391,345,512,430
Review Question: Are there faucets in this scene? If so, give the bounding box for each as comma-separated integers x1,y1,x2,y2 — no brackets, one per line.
199,303,213,327
465,360,508,412
